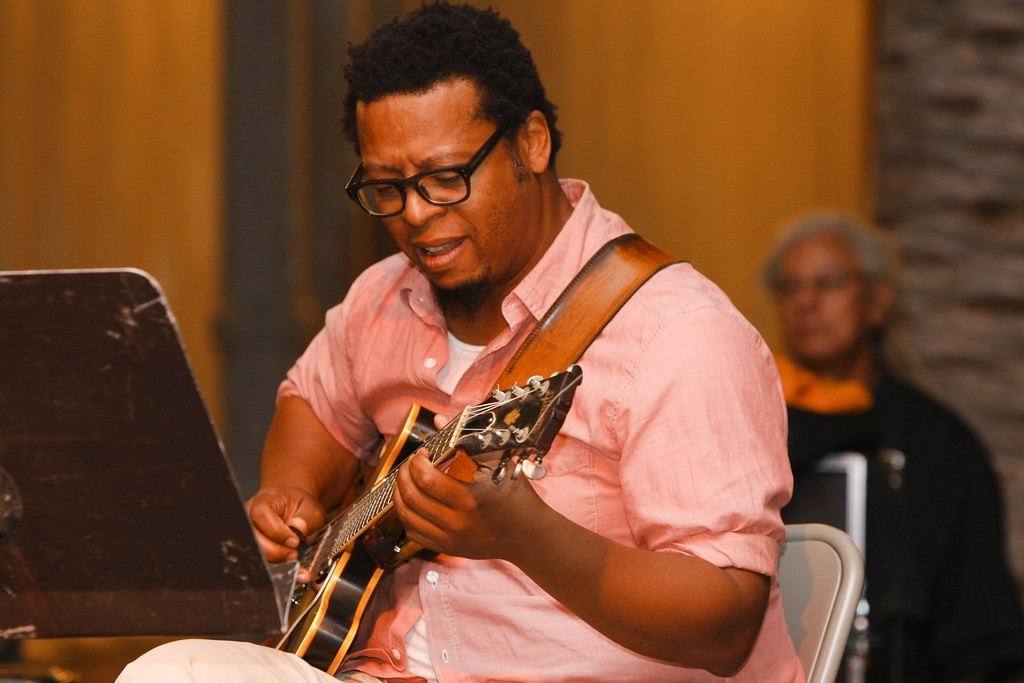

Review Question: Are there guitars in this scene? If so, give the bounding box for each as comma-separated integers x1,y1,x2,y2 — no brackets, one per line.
267,363,584,677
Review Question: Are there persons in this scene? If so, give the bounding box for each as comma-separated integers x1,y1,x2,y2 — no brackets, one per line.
110,0,804,683
762,214,1024,683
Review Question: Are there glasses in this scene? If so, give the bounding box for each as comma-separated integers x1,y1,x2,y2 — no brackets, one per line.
345,123,510,218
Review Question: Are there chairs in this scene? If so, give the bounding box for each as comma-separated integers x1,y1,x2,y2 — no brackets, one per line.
779,523,865,683
